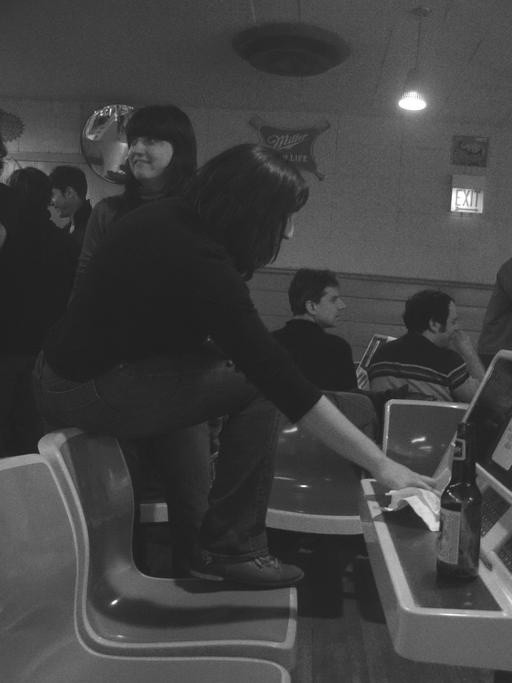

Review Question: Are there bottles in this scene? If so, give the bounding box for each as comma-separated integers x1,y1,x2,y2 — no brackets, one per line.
434,422,486,582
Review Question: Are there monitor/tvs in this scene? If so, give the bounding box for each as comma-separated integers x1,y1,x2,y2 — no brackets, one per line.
450,349,512,506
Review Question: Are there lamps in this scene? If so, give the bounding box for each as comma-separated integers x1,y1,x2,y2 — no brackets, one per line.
399,6,433,112
231,23,350,78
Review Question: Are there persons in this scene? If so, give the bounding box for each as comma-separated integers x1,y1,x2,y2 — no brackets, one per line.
477,257,512,371
270,269,360,393
0,104,210,576
366,289,486,425
32,143,442,591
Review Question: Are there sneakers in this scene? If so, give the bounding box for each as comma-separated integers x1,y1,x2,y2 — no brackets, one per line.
191,555,304,586
